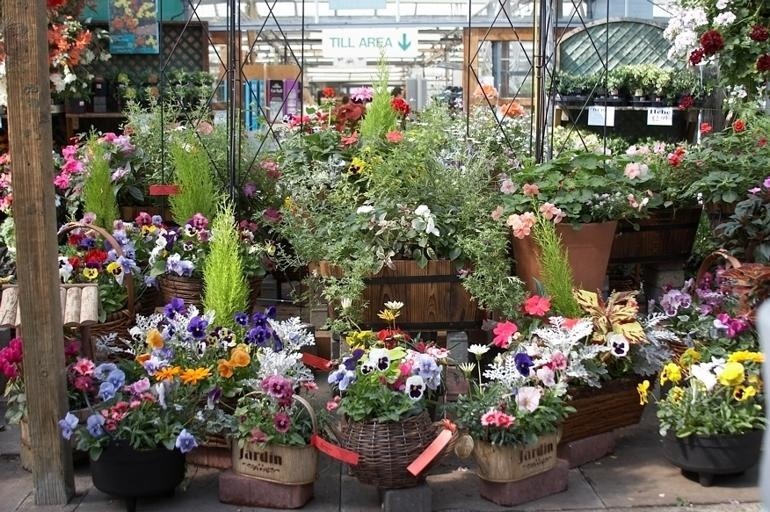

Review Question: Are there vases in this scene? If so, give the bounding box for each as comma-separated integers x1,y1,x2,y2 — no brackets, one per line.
472,418,557,483
227,391,320,485
659,423,763,486
90,441,187,497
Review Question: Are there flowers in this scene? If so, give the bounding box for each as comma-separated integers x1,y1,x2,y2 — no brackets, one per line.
1,2,770,461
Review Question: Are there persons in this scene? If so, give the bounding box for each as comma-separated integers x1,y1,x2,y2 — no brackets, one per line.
392,86,412,118
